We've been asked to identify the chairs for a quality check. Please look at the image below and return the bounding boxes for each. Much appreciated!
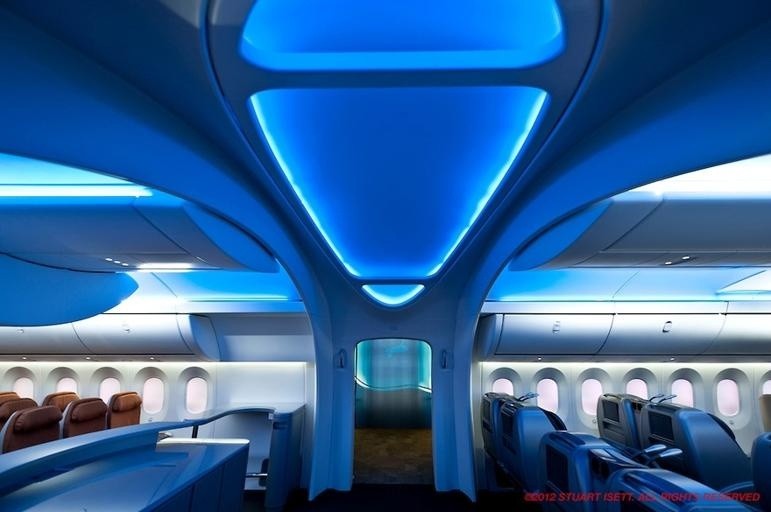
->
[0,391,142,454]
[480,393,771,512]
